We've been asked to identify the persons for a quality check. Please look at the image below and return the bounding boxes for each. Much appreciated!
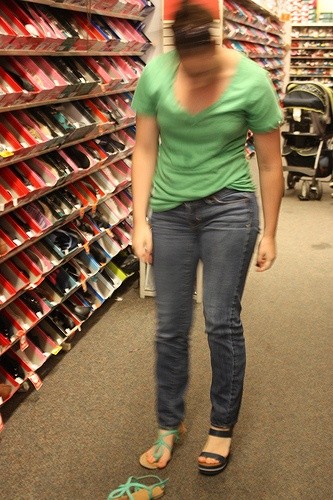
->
[130,4,285,473]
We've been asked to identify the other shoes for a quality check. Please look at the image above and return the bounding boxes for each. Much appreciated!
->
[224,1,333,160]
[0,0,151,405]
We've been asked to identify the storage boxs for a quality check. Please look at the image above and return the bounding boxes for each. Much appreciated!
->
[0,0,153,417]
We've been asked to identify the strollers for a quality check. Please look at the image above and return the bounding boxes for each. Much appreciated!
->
[279,80,333,200]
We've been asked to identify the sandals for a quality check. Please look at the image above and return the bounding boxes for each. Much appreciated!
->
[198,425,235,475]
[106,473,171,500]
[138,426,181,469]
